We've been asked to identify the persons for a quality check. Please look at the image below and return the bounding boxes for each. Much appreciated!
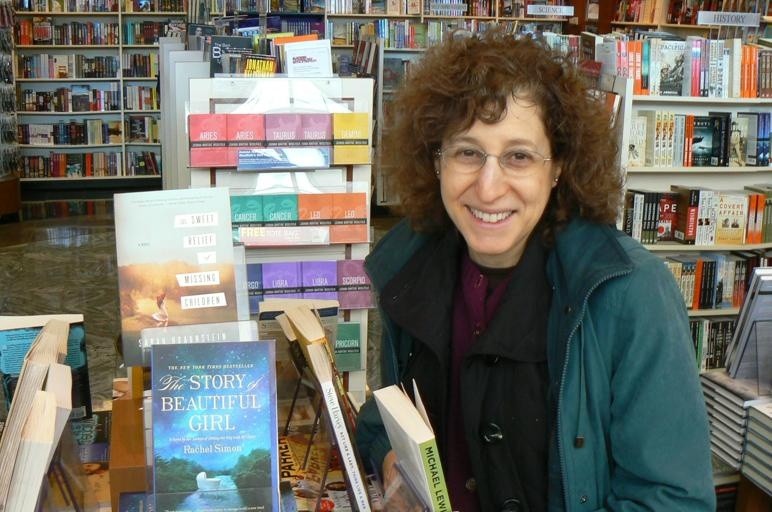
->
[362,34,717,510]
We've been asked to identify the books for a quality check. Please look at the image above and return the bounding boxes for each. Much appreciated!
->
[370,375,456,511]
[116,310,371,511]
[1,1,165,180]
[1,309,109,511]
[370,1,772,310]
[696,309,772,499]
[160,1,377,305]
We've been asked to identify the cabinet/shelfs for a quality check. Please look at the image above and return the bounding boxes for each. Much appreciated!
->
[156,0,772,427]
[0,0,185,183]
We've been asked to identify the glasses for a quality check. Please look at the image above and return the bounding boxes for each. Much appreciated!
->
[439,145,554,176]
